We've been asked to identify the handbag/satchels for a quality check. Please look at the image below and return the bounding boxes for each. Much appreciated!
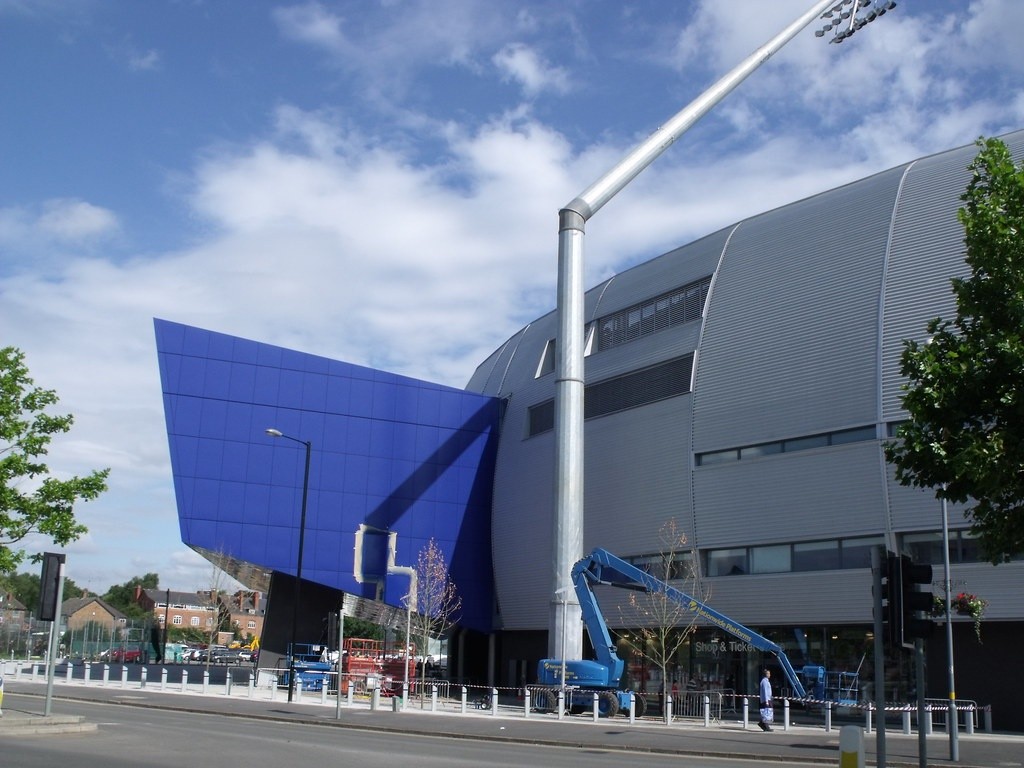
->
[760,707,774,723]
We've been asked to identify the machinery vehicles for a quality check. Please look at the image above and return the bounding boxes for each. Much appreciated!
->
[227,633,259,651]
[533,546,807,718]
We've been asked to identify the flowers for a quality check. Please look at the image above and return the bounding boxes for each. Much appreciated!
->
[929,594,955,617]
[957,586,990,647]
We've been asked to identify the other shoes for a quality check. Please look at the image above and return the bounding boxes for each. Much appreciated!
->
[764,727,774,732]
[758,721,767,731]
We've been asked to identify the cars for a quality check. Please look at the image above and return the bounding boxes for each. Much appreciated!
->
[98,644,142,662]
[181,645,259,664]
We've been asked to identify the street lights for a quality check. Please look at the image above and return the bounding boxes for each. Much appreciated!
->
[264,429,311,700]
[550,0,900,663]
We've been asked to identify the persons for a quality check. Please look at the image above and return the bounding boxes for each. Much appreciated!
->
[672,679,679,714]
[758,670,774,731]
[657,679,668,715]
[305,646,324,661]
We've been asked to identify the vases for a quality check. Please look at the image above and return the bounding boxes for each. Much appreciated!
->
[958,606,970,616]
[933,600,946,610]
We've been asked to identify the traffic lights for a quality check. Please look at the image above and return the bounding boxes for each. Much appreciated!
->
[871,550,935,653]
